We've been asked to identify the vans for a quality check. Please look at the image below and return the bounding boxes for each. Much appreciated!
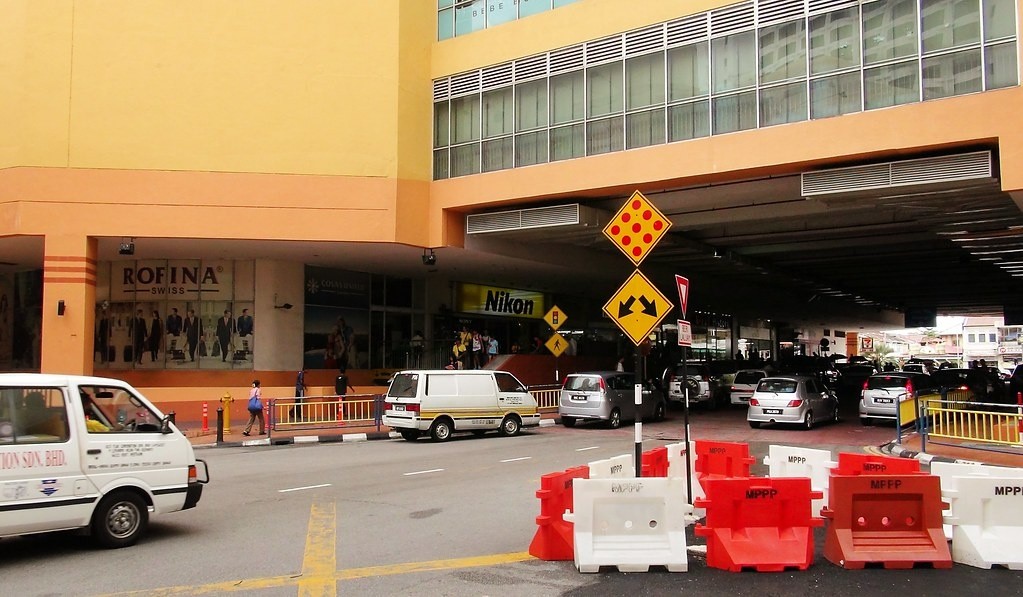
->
[0,372,209,548]
[559,371,668,429]
[381,370,541,442]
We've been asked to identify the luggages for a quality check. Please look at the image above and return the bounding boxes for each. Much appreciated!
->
[105,339,115,362]
[124,339,133,362]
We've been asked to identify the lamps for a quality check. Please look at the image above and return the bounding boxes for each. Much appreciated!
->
[58,300,66,315]
[119,237,134,255]
[422,248,436,266]
[275,303,293,309]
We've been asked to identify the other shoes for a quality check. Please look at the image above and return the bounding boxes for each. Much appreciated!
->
[242,432,250,436]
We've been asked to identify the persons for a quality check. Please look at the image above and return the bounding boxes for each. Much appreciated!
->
[147,310,163,362]
[410,331,424,368]
[237,309,254,360]
[98,309,112,364]
[335,368,355,420]
[289,367,310,418]
[324,316,354,370]
[443,325,987,420]
[183,310,204,362]
[216,310,236,362]
[166,307,182,335]
[242,380,266,436]
[0,391,137,443]
[128,310,146,368]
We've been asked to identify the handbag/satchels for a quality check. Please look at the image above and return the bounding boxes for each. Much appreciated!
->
[211,340,220,356]
[466,343,473,351]
[200,340,207,356]
[248,388,263,411]
[458,350,465,361]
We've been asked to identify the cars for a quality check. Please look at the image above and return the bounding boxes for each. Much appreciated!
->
[730,355,877,430]
[860,358,1023,429]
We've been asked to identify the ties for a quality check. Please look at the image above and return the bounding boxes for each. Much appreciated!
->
[191,318,193,325]
[225,318,227,327]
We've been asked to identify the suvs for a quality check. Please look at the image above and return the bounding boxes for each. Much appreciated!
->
[667,357,737,410]
